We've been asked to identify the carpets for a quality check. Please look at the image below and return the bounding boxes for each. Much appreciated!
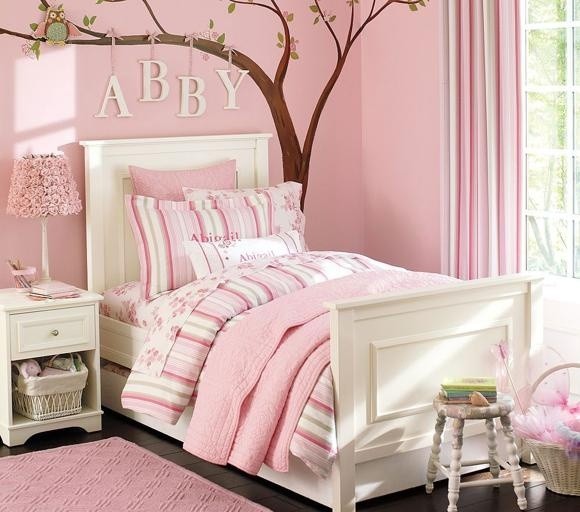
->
[0,435,275,512]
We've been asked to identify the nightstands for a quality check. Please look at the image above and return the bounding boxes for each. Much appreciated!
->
[0,282,107,447]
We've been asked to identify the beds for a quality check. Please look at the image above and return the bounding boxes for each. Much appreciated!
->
[86,267,546,511]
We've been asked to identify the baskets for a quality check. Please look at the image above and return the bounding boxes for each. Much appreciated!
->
[12,353,89,421]
[519,363,580,497]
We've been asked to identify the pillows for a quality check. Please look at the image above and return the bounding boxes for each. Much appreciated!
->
[121,151,309,302]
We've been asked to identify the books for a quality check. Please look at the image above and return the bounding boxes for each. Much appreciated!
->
[30,281,77,296]
[440,377,498,405]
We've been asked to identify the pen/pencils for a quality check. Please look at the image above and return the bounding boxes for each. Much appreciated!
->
[6,259,31,288]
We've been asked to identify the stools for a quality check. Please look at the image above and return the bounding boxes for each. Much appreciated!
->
[424,396,528,512]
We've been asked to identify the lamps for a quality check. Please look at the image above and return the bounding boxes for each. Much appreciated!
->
[5,151,83,295]
